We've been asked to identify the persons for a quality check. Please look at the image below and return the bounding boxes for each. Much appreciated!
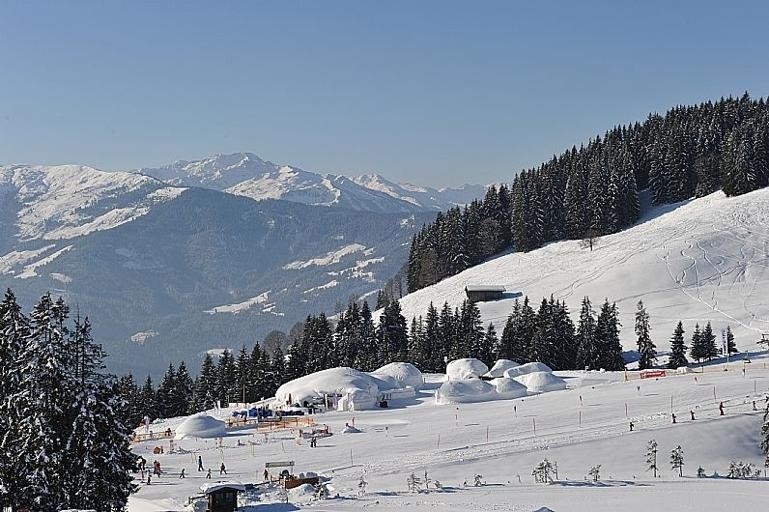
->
[206,467,212,478]
[630,422,634,430]
[136,457,163,484]
[220,462,227,475]
[310,436,314,447]
[262,468,268,480]
[180,467,186,477]
[314,436,317,447]
[197,455,205,471]
[672,413,677,423]
[719,401,726,415]
[690,410,696,420]
